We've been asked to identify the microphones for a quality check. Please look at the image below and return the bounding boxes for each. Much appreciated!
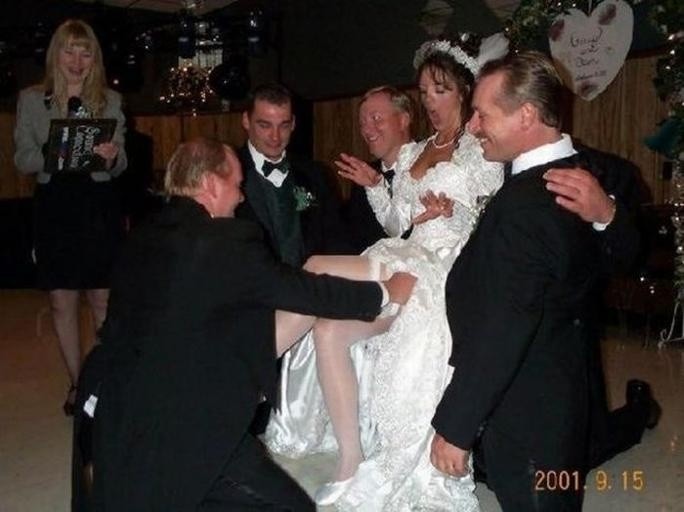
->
[67,96,82,118]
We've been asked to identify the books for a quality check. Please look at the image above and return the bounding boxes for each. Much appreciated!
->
[42,117,118,176]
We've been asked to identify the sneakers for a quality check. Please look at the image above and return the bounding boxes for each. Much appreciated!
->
[315,473,357,508]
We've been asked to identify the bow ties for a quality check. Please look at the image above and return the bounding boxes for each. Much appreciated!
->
[262,157,295,175]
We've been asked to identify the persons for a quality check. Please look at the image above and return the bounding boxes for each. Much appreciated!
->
[232,84,351,270]
[543,50,662,470]
[257,32,505,512]
[430,53,617,512]
[351,81,416,252]
[15,19,129,416]
[71,136,418,512]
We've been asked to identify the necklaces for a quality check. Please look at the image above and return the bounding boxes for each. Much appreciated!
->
[432,130,465,149]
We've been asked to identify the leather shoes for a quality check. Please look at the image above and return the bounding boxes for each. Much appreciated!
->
[627,378,663,426]
[61,385,77,418]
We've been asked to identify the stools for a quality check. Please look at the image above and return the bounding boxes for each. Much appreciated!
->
[604,272,678,352]
